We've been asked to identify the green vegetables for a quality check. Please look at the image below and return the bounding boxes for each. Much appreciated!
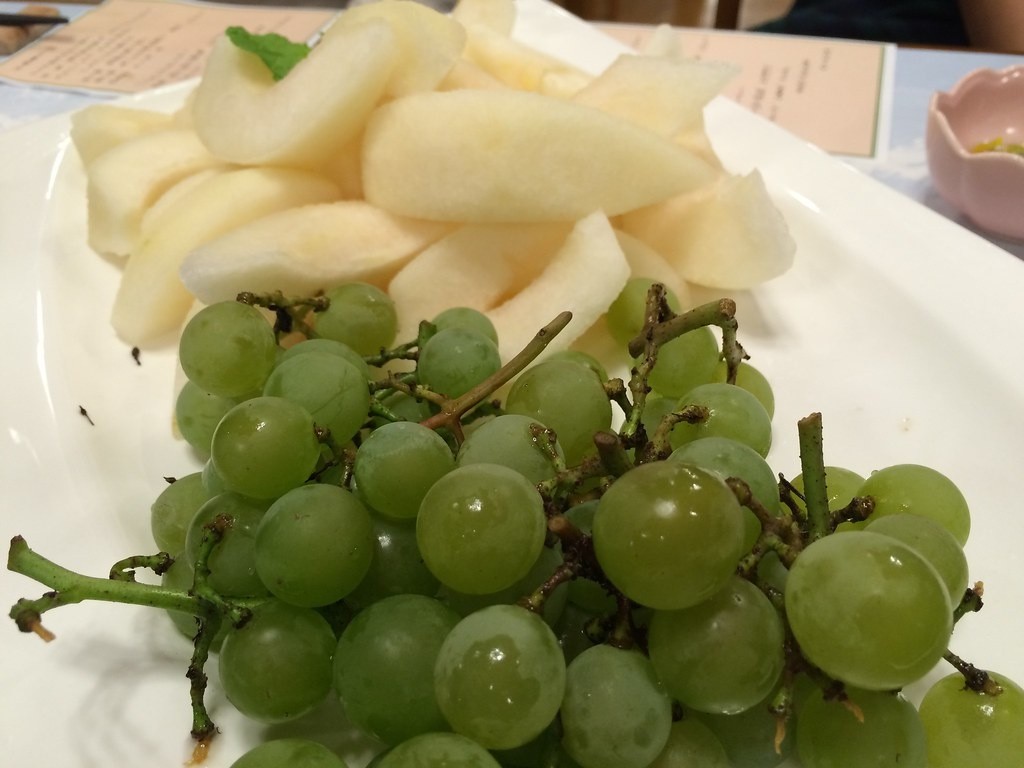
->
[226,25,309,83]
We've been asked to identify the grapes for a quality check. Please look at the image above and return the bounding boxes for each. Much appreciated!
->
[7,272,1024,768]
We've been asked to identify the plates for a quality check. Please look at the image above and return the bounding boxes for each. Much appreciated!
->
[0,0,1024,768]
